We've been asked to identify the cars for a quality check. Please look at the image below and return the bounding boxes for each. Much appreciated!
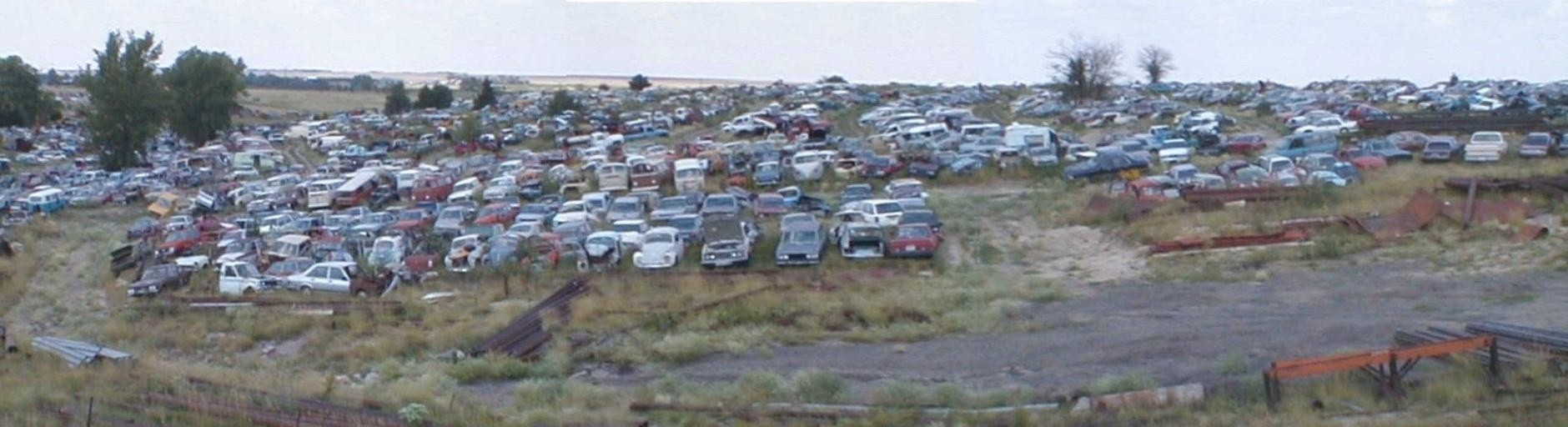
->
[0,94,118,226]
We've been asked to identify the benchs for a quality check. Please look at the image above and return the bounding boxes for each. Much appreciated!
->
[1264,333,1496,406]
